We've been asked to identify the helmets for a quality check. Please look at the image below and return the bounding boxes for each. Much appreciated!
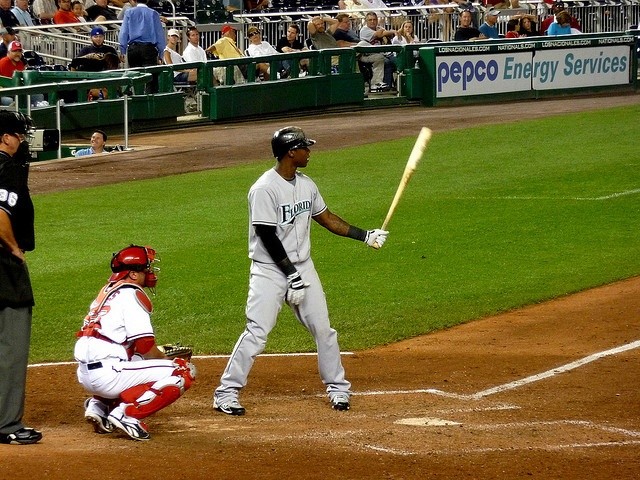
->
[271,126,316,154]
[1,111,38,154]
[108,244,160,296]
[90,28,103,35]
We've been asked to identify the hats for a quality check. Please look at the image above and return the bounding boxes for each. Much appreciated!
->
[8,41,22,51]
[485,7,501,17]
[168,29,180,38]
[222,25,235,33]
[0,26,15,35]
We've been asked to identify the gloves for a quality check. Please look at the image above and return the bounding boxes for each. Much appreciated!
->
[364,229,389,250]
[286,272,310,306]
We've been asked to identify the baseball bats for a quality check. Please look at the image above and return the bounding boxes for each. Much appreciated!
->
[373,126,433,250]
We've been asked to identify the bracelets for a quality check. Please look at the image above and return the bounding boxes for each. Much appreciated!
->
[345,225,367,242]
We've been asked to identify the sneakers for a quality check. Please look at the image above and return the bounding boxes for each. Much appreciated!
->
[213,389,245,416]
[83,397,117,434]
[1,428,41,445]
[330,387,351,410]
[371,82,387,93]
[281,65,291,79]
[108,406,150,440]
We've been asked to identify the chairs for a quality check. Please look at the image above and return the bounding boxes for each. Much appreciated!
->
[163,59,199,112]
[53,64,67,70]
[304,37,339,74]
[425,38,444,43]
[544,29,548,35]
[244,45,277,82]
[24,66,37,73]
[40,64,53,70]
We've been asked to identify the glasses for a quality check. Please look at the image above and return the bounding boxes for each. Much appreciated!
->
[187,27,196,31]
[343,21,350,23]
[12,51,21,54]
[367,19,376,21]
[248,32,258,36]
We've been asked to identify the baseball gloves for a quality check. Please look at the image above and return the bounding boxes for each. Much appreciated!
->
[162,345,193,364]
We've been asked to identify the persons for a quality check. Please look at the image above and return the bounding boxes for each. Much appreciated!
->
[68,26,117,60]
[33,0,59,19]
[118,0,166,94]
[163,29,196,85]
[454,10,487,40]
[70,1,106,23]
[212,127,390,415]
[70,53,118,72]
[0,110,43,445]
[0,26,15,59]
[338,0,371,18]
[75,130,107,158]
[392,20,420,58]
[74,245,196,441]
[205,25,272,79]
[0,41,25,77]
[334,14,389,92]
[478,7,501,39]
[276,25,309,74]
[547,11,572,35]
[505,19,528,39]
[519,15,541,36]
[360,11,401,90]
[0,0,20,34]
[540,2,580,35]
[54,0,87,32]
[247,26,307,78]
[307,17,362,74]
[183,26,218,85]
[83,0,118,30]
[10,0,34,26]
[359,0,392,25]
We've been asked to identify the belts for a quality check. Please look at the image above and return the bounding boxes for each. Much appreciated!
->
[131,43,150,47]
[87,362,103,371]
